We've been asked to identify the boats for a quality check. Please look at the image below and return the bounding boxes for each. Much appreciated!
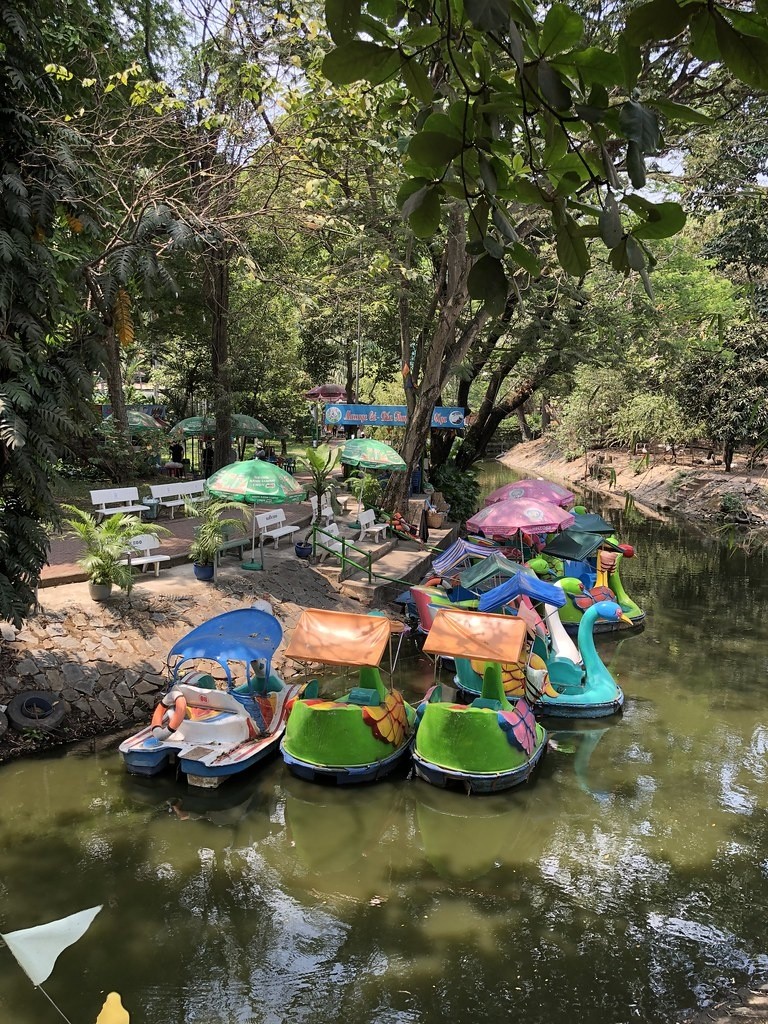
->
[414,609,550,796]
[115,607,301,789]
[409,478,647,720]
[285,606,420,787]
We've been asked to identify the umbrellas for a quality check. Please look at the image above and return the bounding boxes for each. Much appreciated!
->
[305,384,347,404]
[485,479,574,546]
[202,457,307,562]
[169,416,216,437]
[103,410,162,445]
[466,494,575,565]
[338,437,407,521]
[231,413,271,438]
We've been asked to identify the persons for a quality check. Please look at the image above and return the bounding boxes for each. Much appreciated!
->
[169,441,184,477]
[254,441,265,457]
[204,442,214,479]
[332,425,336,435]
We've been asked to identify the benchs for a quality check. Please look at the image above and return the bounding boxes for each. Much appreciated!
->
[150,479,214,520]
[255,508,301,550]
[193,522,250,567]
[358,509,390,543]
[318,523,354,565]
[309,494,336,527]
[89,487,150,524]
[91,533,170,577]
[326,490,349,515]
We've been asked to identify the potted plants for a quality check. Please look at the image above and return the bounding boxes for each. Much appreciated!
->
[178,492,255,581]
[45,503,175,601]
[295,444,347,559]
